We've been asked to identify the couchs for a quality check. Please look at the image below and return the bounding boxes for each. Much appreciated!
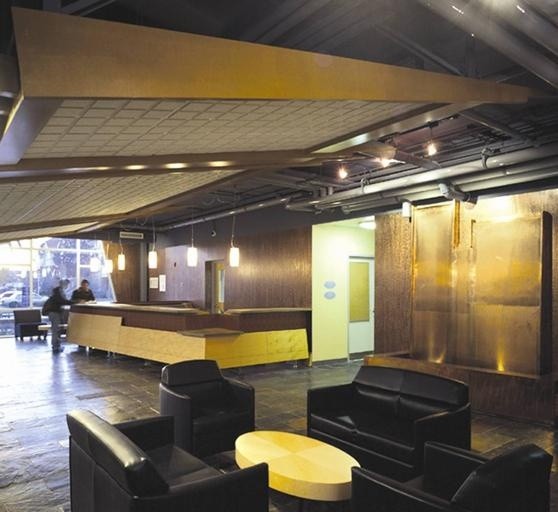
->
[159,360,256,458]
[352,441,553,512]
[13,310,48,341]
[306,365,472,484]
[66,410,269,512]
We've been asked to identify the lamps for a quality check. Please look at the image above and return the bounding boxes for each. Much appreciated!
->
[187,204,198,267]
[377,133,400,168]
[426,123,438,156]
[148,215,158,269]
[229,194,239,268]
[338,163,348,179]
[117,229,126,271]
[358,221,376,231]
[103,231,113,273]
[90,231,100,272]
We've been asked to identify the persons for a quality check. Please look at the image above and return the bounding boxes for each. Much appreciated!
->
[71,280,96,303]
[42,287,78,352]
